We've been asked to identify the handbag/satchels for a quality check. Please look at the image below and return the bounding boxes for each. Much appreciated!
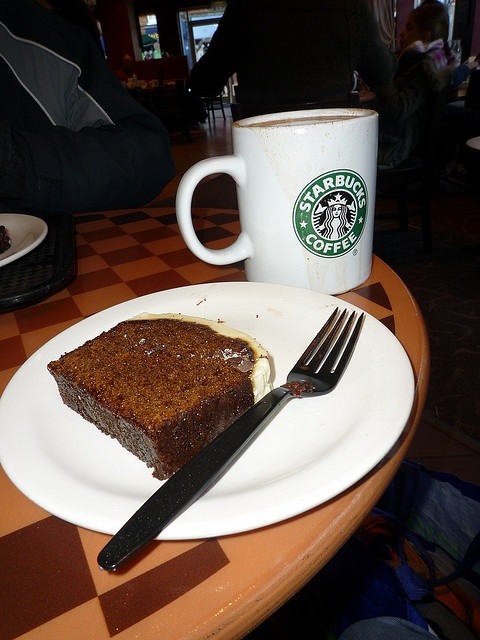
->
[375,456,480,586]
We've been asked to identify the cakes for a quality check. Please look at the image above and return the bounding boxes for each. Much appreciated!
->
[0,220,12,256]
[43,309,274,482]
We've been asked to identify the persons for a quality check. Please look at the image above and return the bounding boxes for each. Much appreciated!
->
[359,0,447,201]
[110,53,133,84]
[433,54,480,191]
[181,0,394,109]
[1,2,177,213]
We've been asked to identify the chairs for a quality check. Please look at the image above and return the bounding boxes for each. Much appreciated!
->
[376,79,448,252]
[231,92,364,122]
[201,93,226,124]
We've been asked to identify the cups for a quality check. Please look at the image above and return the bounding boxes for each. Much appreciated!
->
[176,107,380,296]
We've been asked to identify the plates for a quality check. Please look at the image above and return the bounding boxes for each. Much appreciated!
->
[1,212,48,268]
[0,281,415,542]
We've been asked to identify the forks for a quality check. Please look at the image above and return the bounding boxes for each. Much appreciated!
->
[97,308,364,568]
